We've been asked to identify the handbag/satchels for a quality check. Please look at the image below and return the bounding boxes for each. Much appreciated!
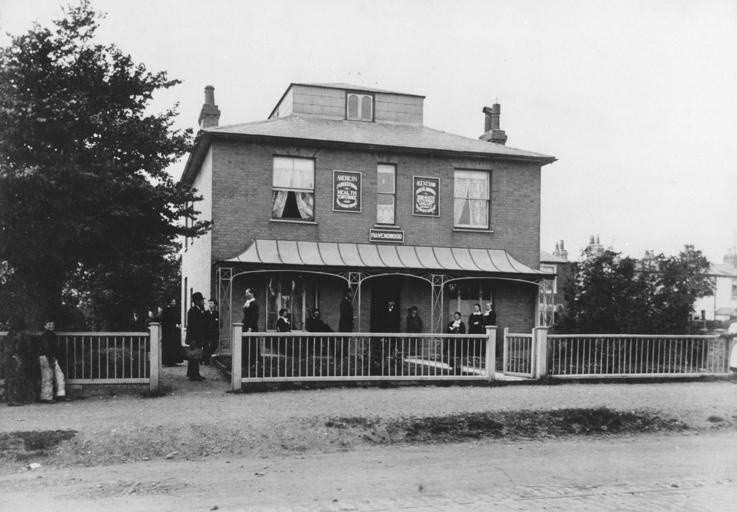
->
[183,345,203,360]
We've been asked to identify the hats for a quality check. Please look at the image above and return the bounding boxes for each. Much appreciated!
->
[191,292,205,302]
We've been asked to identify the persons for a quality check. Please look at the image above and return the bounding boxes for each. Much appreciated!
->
[240,287,354,370]
[161,292,223,380]
[34,316,73,403]
[385,298,423,355]
[444,303,497,359]
[3,317,32,407]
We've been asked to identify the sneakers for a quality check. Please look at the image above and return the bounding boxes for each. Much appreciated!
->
[190,373,205,381]
[41,399,55,402]
[57,395,71,402]
